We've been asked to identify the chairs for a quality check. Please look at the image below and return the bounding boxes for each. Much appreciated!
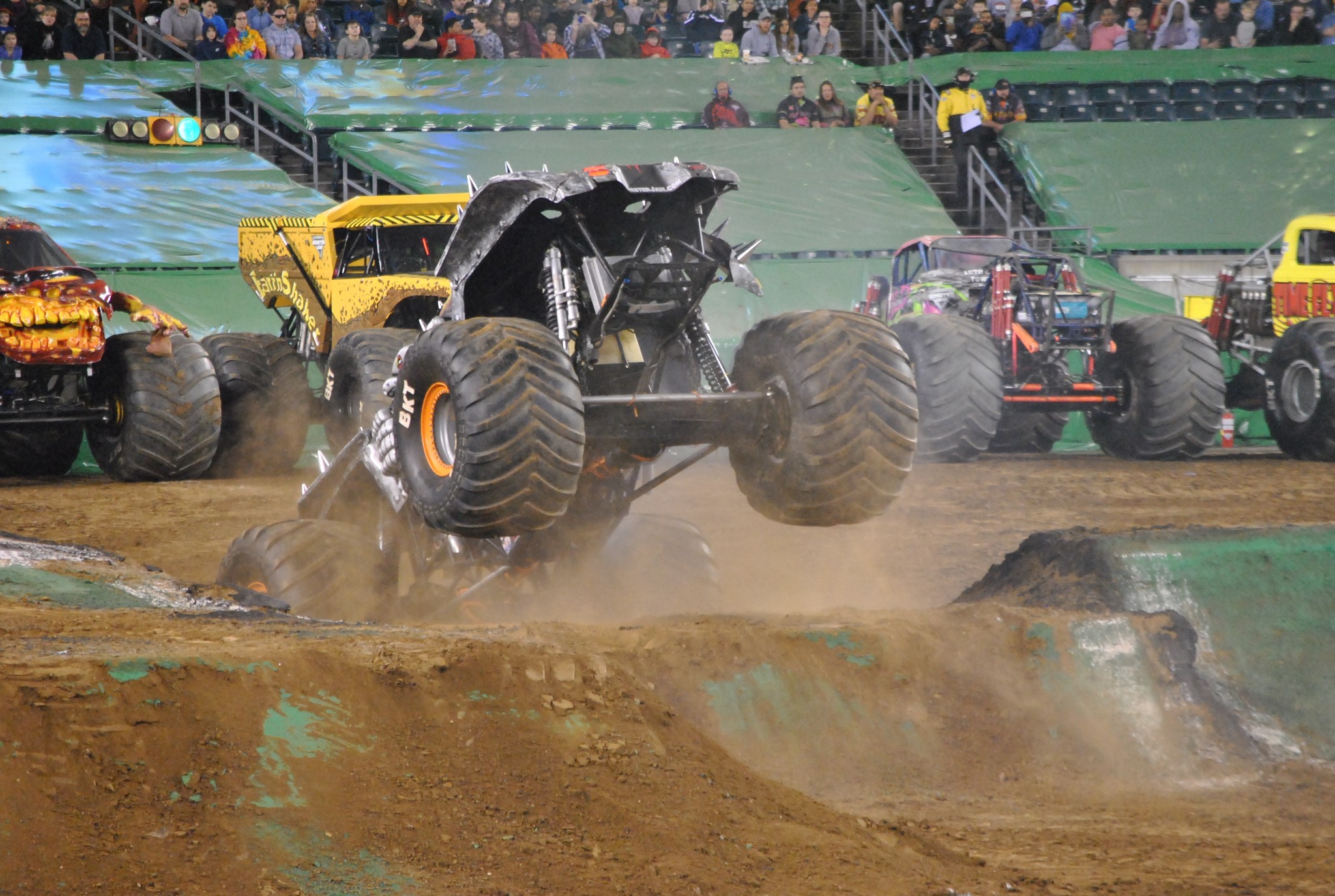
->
[317,1,399,60]
[627,24,718,59]
[980,76,1335,123]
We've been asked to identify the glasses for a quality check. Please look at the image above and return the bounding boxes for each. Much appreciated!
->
[816,16,831,20]
[870,82,883,87]
[271,14,286,19]
[236,17,246,21]
[577,12,586,15]
[347,25,360,30]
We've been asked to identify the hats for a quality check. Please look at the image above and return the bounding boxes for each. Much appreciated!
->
[408,7,422,17]
[957,67,971,75]
[576,4,590,13]
[869,80,884,88]
[995,79,1011,89]
[758,12,771,20]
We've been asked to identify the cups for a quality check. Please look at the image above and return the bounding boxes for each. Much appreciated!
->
[446,38,457,52]
[742,49,750,58]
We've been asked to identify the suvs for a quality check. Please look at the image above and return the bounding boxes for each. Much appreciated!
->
[849,232,1228,467]
[1195,211,1334,464]
[0,216,220,483]
[212,157,925,625]
[197,191,479,475]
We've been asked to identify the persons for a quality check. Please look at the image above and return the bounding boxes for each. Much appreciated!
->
[776,76,822,128]
[855,81,898,127]
[702,82,751,128]
[936,66,996,208]
[0,0,1335,62]
[982,78,1026,157]
[814,81,851,128]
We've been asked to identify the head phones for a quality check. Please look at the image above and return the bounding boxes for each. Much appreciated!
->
[713,88,732,96]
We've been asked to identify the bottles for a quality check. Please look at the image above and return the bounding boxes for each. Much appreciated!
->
[399,17,404,27]
[887,128,895,140]
[1127,19,1138,32]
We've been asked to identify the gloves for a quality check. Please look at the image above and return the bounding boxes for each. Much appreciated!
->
[942,131,954,149]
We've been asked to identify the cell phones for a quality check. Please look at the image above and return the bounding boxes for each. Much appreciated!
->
[578,16,582,23]
